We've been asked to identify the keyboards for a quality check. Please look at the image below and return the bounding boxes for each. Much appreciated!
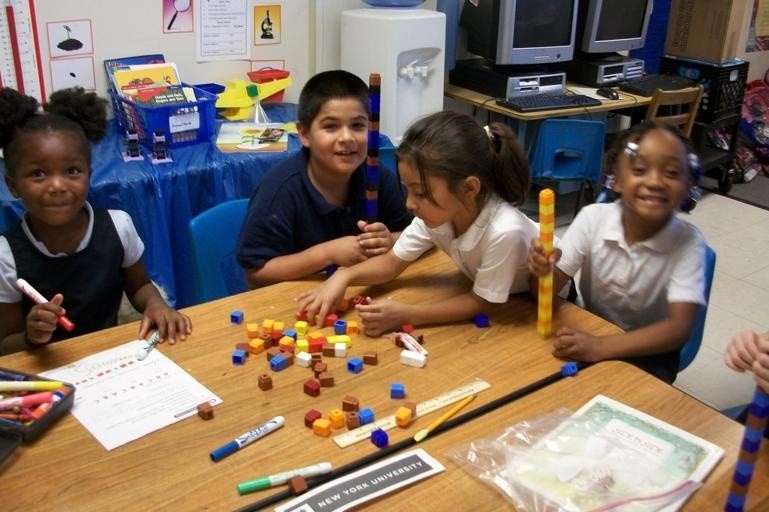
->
[495,94,601,112]
[618,74,696,96]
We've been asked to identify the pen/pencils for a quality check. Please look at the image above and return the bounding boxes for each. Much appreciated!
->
[413,395,477,442]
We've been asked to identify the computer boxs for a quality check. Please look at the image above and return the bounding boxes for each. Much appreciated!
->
[567,55,645,87]
[454,58,567,101]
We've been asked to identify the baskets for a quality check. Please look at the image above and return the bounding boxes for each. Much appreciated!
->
[107,82,219,150]
[661,56,749,123]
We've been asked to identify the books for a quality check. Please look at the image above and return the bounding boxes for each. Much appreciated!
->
[104,53,199,144]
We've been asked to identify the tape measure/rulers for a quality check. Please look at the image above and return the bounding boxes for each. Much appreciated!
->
[332,376,492,449]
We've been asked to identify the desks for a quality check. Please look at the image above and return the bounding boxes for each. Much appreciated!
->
[2,100,412,310]
[256,359,769,511]
[2,247,627,512]
[442,69,697,207]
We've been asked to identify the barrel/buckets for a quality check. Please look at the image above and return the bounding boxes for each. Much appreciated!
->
[361,0,428,9]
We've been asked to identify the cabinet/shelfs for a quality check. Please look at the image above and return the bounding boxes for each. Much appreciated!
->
[660,55,749,176]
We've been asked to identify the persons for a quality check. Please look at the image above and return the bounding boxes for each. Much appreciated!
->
[291,108,579,338]
[723,328,769,441]
[524,117,708,387]
[0,84,193,356]
[234,68,416,291]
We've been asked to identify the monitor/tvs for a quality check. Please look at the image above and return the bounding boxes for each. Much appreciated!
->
[580,0,654,64]
[458,0,579,73]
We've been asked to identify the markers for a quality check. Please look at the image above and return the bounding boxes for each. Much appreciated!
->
[15,278,75,332]
[137,328,161,361]
[0,369,71,427]
[237,462,332,496]
[210,415,286,462]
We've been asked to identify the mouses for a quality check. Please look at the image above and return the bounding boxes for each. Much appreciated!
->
[596,87,618,100]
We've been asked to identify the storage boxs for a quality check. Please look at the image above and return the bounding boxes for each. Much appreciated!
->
[663,0,746,64]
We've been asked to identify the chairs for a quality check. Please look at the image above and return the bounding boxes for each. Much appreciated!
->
[524,118,607,217]
[643,83,705,139]
[675,242,716,372]
[188,196,251,303]
